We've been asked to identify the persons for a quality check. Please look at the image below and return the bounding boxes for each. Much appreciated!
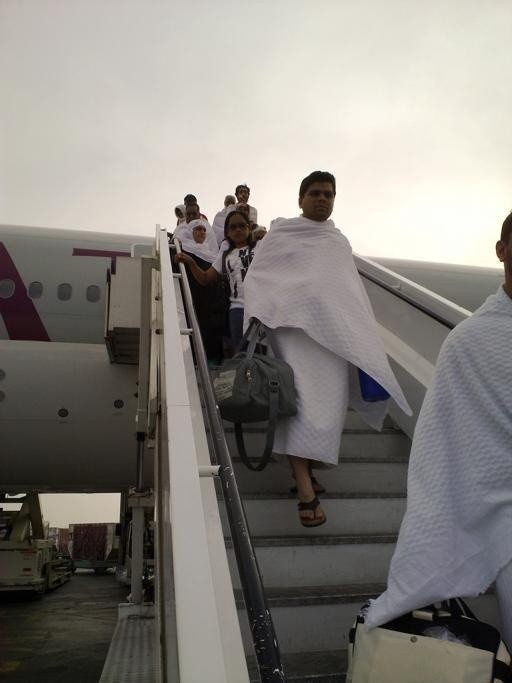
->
[168,185,272,370]
[241,170,412,528]
[366,209,512,683]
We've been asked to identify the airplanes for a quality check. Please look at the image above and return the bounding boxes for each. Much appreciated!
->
[0,224,512,681]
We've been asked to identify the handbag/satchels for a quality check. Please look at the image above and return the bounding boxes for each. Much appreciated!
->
[343,592,512,682]
[213,317,299,424]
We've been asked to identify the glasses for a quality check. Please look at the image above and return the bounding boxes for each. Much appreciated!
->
[228,222,250,230]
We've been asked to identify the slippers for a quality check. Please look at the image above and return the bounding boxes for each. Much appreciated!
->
[290,475,326,493]
[297,492,327,527]
